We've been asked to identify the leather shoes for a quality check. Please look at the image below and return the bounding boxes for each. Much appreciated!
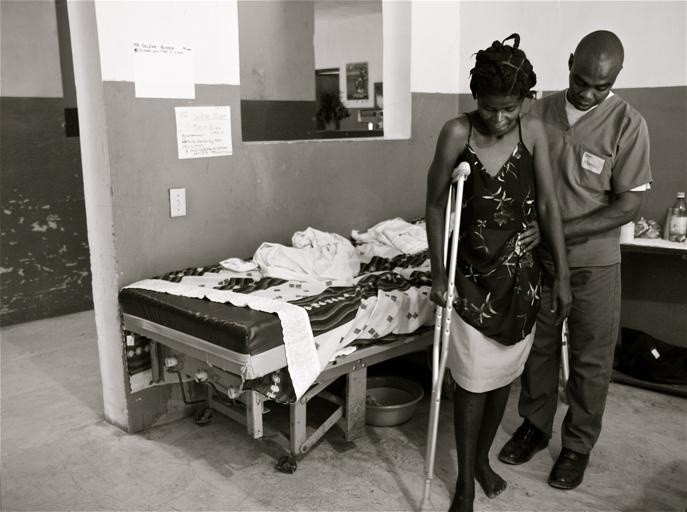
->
[497,422,551,466]
[545,447,591,488]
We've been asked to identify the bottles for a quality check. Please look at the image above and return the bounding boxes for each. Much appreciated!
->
[669,192,685,242]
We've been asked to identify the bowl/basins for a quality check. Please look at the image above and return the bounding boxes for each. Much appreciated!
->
[364,378,423,428]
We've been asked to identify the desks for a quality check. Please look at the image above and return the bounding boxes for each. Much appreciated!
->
[609,239,687,399]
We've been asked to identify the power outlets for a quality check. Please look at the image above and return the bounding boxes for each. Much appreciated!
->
[169,188,186,218]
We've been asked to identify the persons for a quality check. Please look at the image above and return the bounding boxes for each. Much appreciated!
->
[426,34,573,512]
[497,31,653,490]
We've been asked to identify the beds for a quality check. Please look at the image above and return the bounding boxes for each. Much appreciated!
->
[118,216,441,474]
